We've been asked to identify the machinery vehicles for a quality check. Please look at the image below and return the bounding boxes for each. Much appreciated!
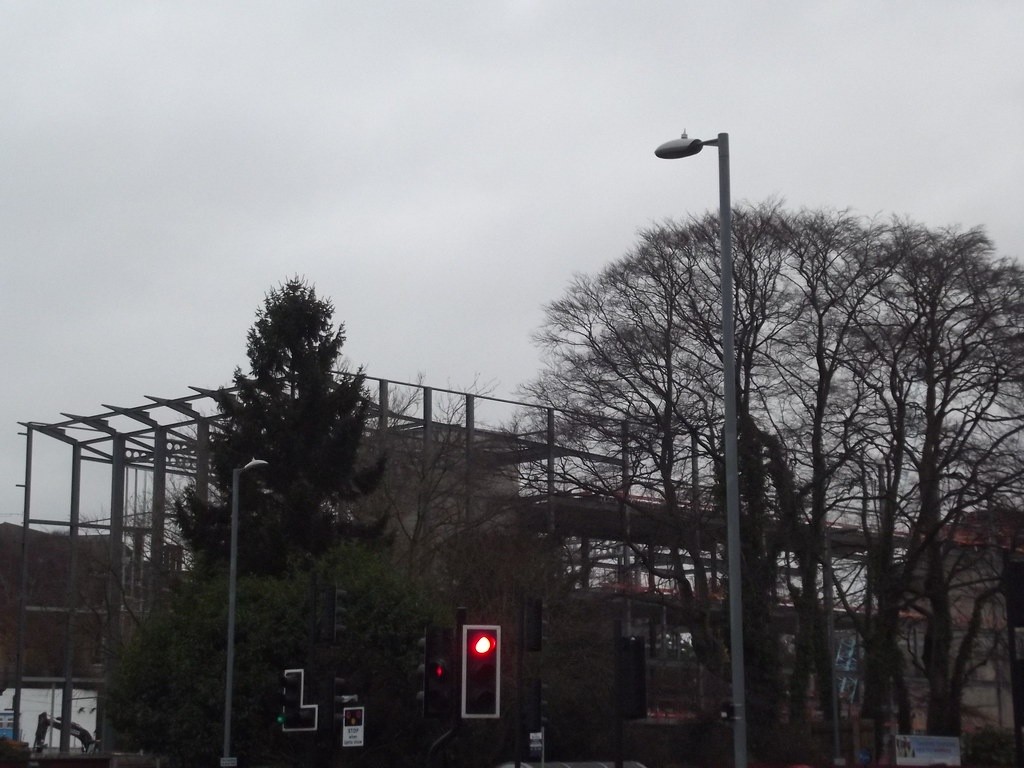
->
[34,712,98,758]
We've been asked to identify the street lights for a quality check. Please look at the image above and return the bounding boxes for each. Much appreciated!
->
[220,456,269,768]
[655,126,755,768]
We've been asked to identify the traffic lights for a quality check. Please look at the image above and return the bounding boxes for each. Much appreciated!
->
[322,586,348,640]
[424,624,457,722]
[278,668,347,737]
[461,622,502,719]
